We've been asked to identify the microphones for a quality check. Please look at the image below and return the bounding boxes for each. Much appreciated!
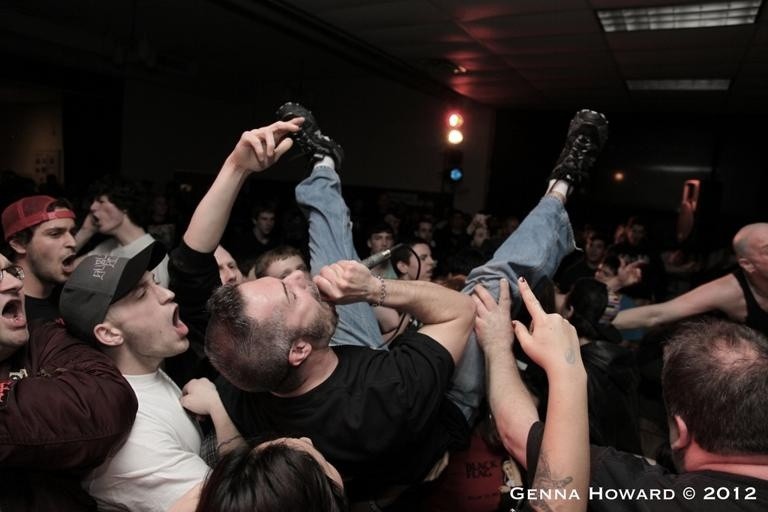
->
[316,242,407,296]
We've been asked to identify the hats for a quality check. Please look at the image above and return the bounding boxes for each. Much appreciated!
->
[59,240,165,340]
[2,198,77,238]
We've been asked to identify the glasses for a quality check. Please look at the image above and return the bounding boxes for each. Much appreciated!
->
[1,266,24,280]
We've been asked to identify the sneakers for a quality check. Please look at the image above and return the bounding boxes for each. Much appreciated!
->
[278,102,344,167]
[549,109,608,192]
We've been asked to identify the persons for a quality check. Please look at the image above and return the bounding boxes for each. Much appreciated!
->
[0,102,768,512]
